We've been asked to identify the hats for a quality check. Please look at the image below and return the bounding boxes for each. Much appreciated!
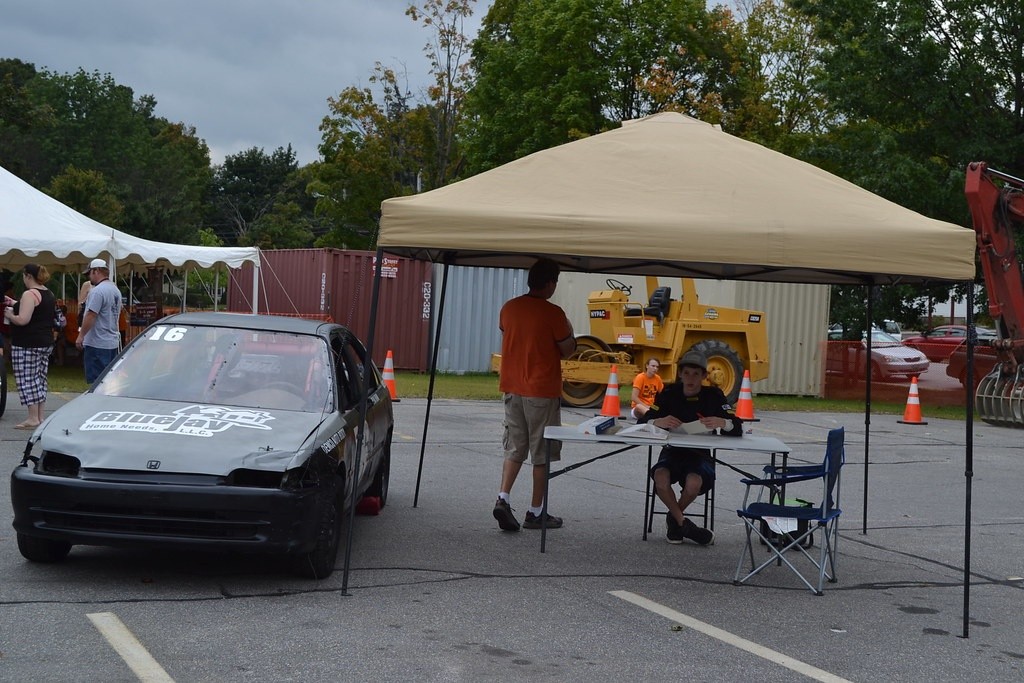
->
[81,259,108,277]
[675,350,709,372]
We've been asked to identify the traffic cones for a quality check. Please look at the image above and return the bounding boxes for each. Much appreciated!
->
[896,376,928,426]
[383,349,401,403]
[731,368,760,423]
[595,364,627,420]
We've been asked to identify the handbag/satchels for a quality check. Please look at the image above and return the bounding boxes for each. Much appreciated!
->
[52,298,66,333]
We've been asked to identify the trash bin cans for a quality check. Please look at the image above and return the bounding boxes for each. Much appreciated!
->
[129,312,149,342]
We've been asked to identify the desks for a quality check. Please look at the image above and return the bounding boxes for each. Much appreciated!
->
[541,425,792,566]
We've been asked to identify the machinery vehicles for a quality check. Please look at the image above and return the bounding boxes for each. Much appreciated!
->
[491,277,770,409]
[965,162,1024,426]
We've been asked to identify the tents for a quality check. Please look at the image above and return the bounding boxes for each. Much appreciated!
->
[340,112,977,640]
[0,165,306,342]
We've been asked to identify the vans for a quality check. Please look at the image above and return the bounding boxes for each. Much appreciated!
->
[827,319,902,342]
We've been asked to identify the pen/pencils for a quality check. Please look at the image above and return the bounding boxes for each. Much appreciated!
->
[697,413,704,419]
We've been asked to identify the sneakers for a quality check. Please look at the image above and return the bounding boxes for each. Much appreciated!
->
[665,510,684,545]
[678,519,715,546]
[521,508,563,530]
[493,496,524,532]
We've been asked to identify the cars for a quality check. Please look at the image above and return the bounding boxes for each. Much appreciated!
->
[827,328,930,380]
[10,310,394,580]
[122,298,139,307]
[901,325,990,362]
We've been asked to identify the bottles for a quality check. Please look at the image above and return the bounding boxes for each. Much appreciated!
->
[3,305,13,325]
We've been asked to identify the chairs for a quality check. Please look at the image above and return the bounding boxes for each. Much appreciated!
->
[626,286,671,317]
[733,426,846,595]
[647,448,716,542]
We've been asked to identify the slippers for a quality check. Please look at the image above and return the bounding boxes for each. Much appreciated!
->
[14,421,38,430]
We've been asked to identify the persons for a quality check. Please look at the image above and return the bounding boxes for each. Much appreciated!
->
[74,259,122,384]
[636,351,743,546]
[0,263,56,430]
[630,357,664,420]
[493,258,578,532]
